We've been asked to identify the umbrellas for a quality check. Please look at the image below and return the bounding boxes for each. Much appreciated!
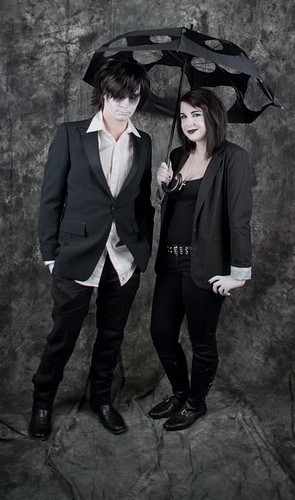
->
[80,25,286,193]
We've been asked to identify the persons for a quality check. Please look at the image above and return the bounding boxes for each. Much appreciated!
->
[28,58,156,442]
[149,88,252,430]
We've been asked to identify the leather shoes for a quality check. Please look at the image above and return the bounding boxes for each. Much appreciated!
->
[148,390,207,432]
[87,394,127,435]
[28,403,53,441]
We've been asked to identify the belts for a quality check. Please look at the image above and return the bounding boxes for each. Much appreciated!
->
[165,244,192,257]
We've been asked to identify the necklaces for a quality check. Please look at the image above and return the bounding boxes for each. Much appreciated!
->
[178,148,205,190]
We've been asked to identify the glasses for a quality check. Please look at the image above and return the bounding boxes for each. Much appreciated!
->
[108,90,141,105]
